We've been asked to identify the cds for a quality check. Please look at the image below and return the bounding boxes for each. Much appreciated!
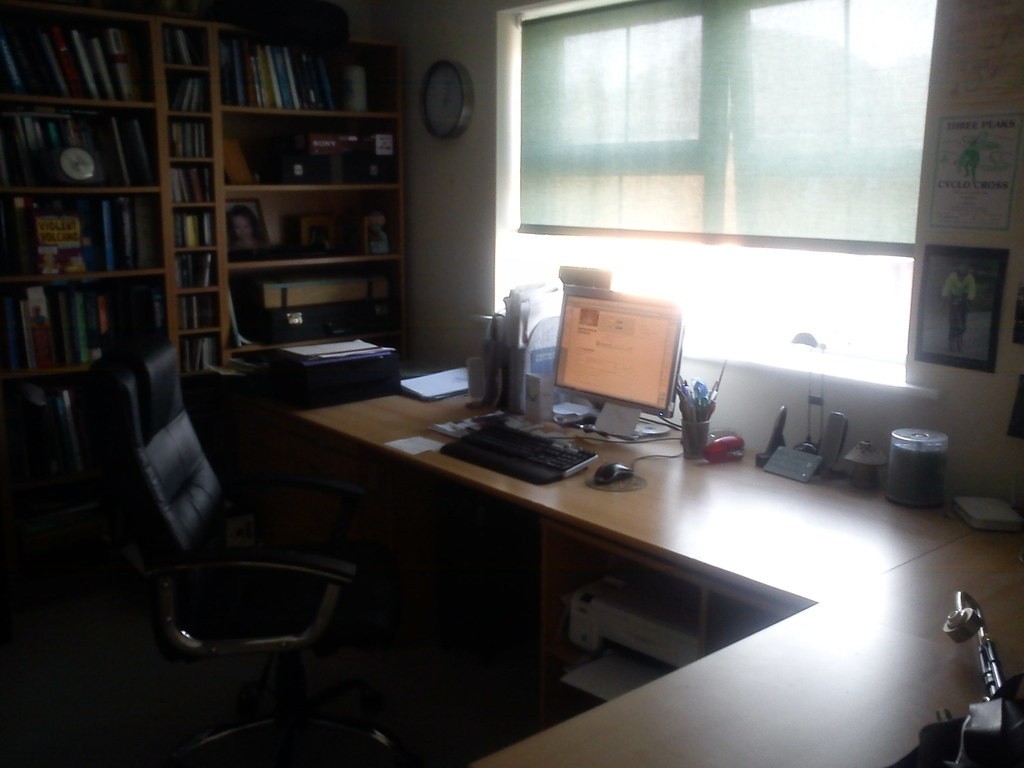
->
[635,423,670,434]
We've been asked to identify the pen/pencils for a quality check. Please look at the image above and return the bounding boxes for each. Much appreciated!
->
[676,363,726,424]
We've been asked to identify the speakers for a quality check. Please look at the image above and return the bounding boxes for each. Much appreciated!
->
[818,412,848,480]
[755,405,789,465]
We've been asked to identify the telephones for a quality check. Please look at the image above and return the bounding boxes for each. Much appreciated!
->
[755,401,788,473]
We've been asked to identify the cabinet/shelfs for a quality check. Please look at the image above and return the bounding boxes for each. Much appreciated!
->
[1,0,408,645]
[538,515,709,730]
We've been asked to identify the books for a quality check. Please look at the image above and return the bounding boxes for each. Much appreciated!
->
[219,37,368,112]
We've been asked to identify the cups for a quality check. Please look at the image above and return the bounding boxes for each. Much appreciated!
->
[681,419,711,459]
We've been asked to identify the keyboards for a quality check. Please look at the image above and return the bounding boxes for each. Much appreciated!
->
[438,423,598,486]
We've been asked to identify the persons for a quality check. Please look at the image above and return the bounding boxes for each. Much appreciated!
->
[941,263,976,352]
[0,27,217,371]
[227,205,268,259]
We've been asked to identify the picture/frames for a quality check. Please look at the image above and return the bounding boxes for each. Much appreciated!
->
[914,244,1010,373]
[224,199,268,250]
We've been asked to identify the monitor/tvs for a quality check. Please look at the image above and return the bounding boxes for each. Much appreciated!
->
[554,285,687,442]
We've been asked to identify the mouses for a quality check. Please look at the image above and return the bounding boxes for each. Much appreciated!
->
[595,462,634,486]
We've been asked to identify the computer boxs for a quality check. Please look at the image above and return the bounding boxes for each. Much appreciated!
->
[433,485,542,661]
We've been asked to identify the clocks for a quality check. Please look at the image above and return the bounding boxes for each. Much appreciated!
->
[418,60,474,139]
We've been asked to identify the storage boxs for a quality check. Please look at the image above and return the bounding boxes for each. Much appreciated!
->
[261,132,397,157]
[231,346,402,409]
[524,372,554,423]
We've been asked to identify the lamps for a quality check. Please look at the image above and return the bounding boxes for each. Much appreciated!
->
[791,333,824,455]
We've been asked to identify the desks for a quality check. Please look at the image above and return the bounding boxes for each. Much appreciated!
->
[234,358,1024,768]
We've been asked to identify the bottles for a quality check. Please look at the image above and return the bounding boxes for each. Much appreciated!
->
[884,426,951,507]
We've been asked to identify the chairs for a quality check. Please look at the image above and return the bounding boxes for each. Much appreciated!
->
[81,332,426,767]
